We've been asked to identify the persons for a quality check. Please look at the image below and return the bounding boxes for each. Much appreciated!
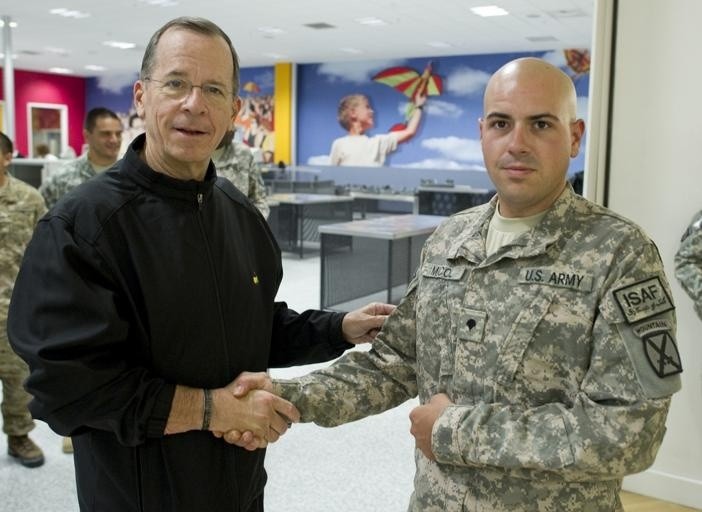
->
[1,133,46,468]
[235,95,274,151]
[210,119,271,222]
[38,145,59,185]
[209,58,686,512]
[6,17,401,512]
[38,108,129,455]
[675,211,701,319]
[325,86,430,172]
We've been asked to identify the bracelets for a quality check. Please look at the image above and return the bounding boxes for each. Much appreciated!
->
[198,384,213,438]
[414,103,425,111]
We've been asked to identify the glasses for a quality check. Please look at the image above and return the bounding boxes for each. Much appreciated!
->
[144,77,234,107]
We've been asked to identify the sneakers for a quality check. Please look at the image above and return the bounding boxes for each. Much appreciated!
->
[61,435,76,455]
[7,433,45,469]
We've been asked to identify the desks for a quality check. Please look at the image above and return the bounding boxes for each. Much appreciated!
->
[260,164,495,259]
[319,215,452,311]
